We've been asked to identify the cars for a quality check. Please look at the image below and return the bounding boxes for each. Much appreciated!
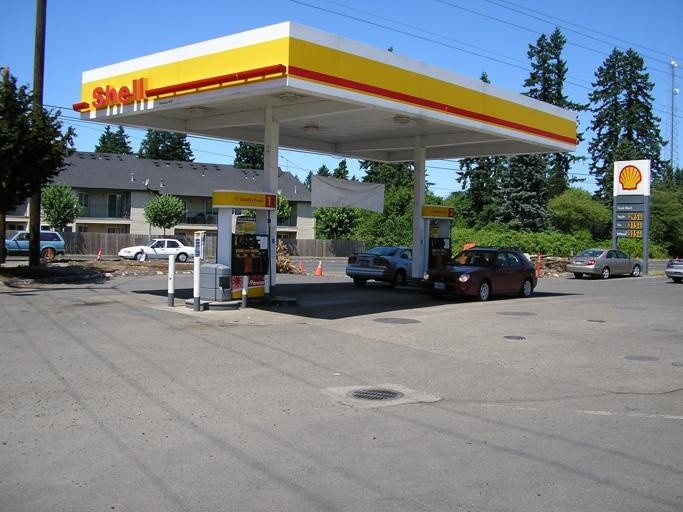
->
[664,258,683,283]
[423,245,538,301]
[117,238,195,263]
[345,245,413,286]
[565,248,642,279]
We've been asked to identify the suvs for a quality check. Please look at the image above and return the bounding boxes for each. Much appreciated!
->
[4,230,67,260]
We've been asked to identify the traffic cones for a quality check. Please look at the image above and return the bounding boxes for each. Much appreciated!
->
[314,259,323,277]
